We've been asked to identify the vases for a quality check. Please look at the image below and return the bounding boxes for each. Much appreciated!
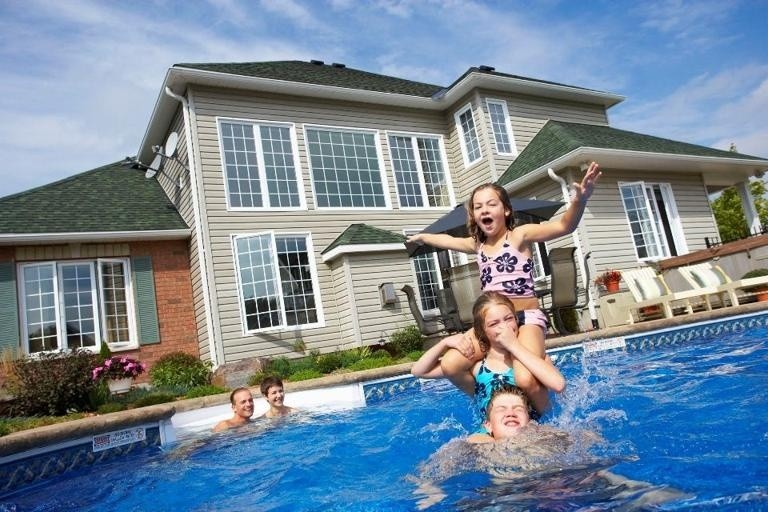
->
[604,281,620,293]
[104,376,134,394]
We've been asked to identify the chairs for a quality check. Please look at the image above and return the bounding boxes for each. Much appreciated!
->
[536,246,593,337]
[401,261,482,337]
[622,261,767,321]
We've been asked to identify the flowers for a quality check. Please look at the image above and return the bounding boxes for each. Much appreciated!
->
[91,354,146,384]
[595,267,621,285]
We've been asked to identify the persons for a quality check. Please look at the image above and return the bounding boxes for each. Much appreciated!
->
[404,160,604,417]
[257,377,317,427]
[172,386,259,458]
[410,291,566,439]
[406,382,692,512]
